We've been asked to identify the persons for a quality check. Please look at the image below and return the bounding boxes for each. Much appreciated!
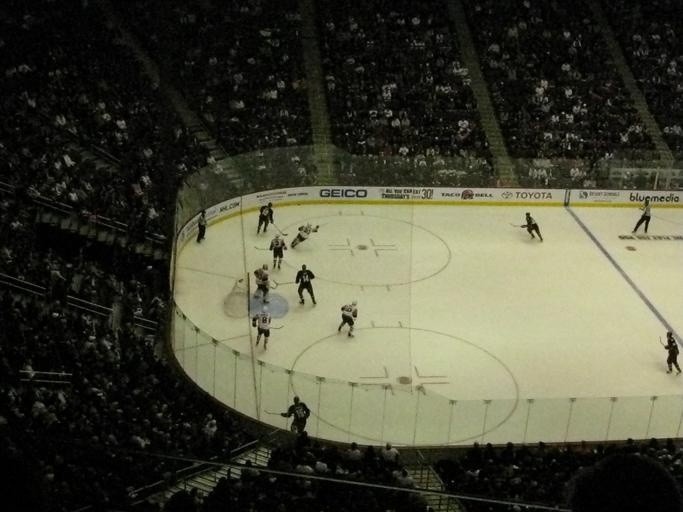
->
[0,189,227,512]
[336,299,358,338]
[268,234,287,269]
[255,201,275,233]
[294,264,317,306]
[251,304,273,350]
[251,263,271,304]
[280,395,311,433]
[227,409,683,511]
[290,220,320,250]
[632,199,652,233]
[664,331,682,374]
[0,0,683,191]
[520,212,543,241]
